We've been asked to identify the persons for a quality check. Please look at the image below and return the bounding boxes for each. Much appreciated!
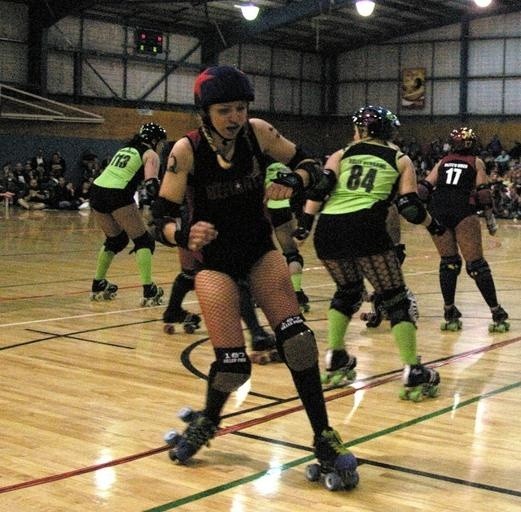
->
[88,123,168,307]
[360,127,521,323]
[289,106,446,387]
[147,65,359,472]
[162,160,311,335]
[0,148,112,212]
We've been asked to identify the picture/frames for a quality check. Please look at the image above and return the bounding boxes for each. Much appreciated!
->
[400,67,425,111]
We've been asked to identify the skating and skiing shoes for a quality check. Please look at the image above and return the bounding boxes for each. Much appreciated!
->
[489,304,510,333]
[164,406,218,465]
[248,334,285,365]
[361,290,381,327]
[319,349,356,387]
[398,356,440,402]
[140,284,202,333]
[441,305,462,331]
[295,289,310,313]
[90,278,118,300]
[305,429,359,491]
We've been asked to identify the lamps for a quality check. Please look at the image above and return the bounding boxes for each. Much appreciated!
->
[355,0,375,17]
[241,6,260,21]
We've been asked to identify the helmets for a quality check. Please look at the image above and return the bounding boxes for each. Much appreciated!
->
[194,65,255,108]
[352,105,401,142]
[140,122,167,144]
[447,125,477,153]
